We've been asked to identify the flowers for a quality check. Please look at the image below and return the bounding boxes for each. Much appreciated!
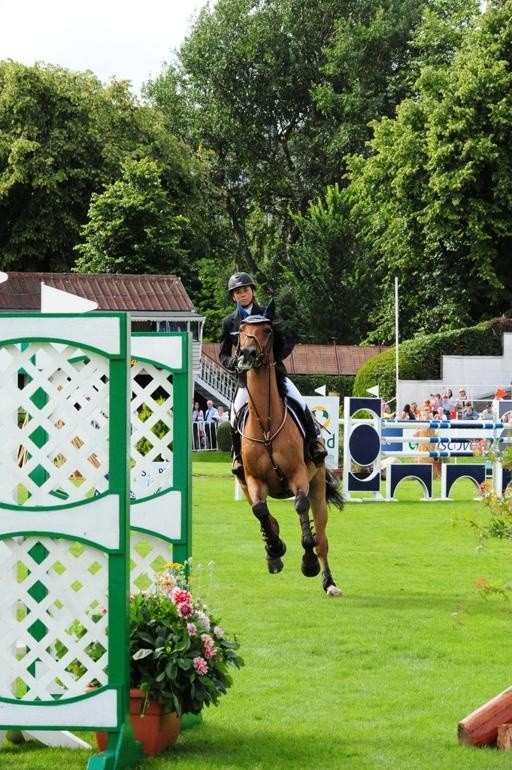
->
[54,557,245,720]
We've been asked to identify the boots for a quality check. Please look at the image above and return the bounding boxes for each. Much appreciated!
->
[227,424,243,475]
[303,404,327,465]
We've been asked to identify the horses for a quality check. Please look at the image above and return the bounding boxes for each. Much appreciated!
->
[231,298,349,599]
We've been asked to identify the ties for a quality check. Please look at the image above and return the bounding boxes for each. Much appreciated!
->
[205,410,211,421]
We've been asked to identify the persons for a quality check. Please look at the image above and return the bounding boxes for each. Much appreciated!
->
[215,273,328,474]
[204,400,218,449]
[380,386,497,420]
[216,405,229,427]
[193,402,205,450]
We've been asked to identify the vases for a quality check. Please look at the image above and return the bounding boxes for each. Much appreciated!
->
[81,685,181,756]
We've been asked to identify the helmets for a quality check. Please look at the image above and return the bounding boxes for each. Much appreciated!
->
[227,272,257,294]
